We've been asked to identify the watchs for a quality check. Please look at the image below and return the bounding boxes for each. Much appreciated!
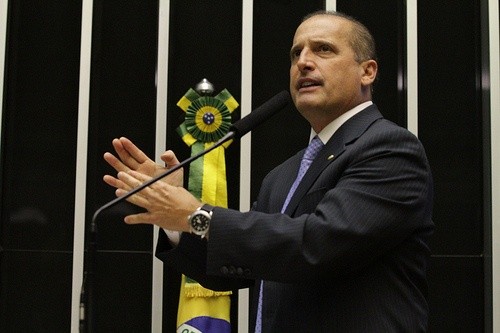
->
[186,202,213,240]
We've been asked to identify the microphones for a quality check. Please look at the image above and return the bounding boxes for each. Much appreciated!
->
[90,88,291,250]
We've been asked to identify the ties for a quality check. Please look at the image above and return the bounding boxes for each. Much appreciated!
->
[254,137,322,332]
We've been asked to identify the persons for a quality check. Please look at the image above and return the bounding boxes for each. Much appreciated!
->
[102,10,433,333]
